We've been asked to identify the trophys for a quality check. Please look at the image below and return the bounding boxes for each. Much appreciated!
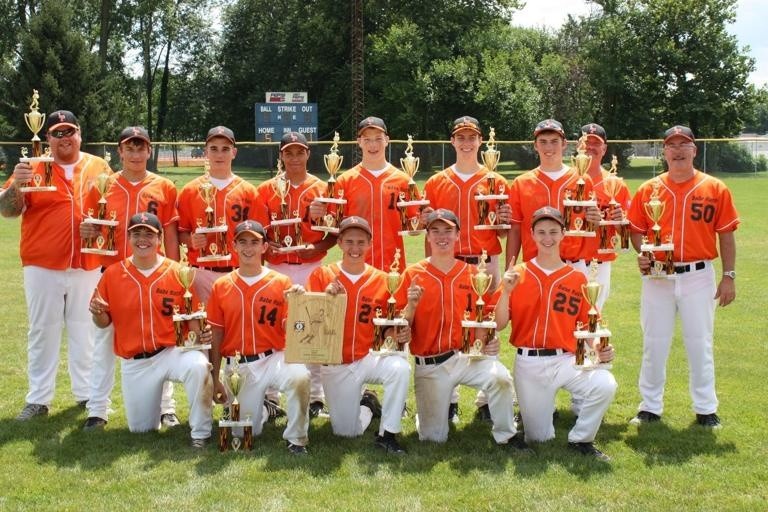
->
[573,257,612,369]
[598,153,630,254]
[171,243,213,350]
[474,125,512,231]
[370,248,410,357]
[17,87,58,192]
[216,350,254,452]
[79,152,120,256]
[461,248,497,360]
[396,131,431,236]
[312,130,348,234]
[194,159,232,262]
[269,158,306,253]
[563,131,597,238]
[640,175,678,281]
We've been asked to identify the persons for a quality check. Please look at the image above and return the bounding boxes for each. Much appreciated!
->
[627,124,740,431]
[82,127,182,428]
[489,205,619,463]
[572,123,633,346]
[90,215,216,449]
[303,216,413,454]
[177,127,270,359]
[502,118,597,282]
[388,209,533,456]
[420,116,510,294]
[257,132,332,417]
[309,116,435,274]
[206,220,310,454]
[1,110,116,421]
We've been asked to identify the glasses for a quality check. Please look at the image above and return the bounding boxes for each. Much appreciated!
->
[52,127,76,138]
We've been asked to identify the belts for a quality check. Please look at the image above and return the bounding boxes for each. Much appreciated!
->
[411,348,465,366]
[226,347,287,366]
[129,344,169,362]
[452,253,495,267]
[640,260,712,273]
[515,344,578,357]
[191,264,242,273]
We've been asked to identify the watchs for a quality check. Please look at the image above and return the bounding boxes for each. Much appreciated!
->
[723,270,736,279]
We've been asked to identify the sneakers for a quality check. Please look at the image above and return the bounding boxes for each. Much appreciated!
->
[696,409,724,430]
[480,403,492,425]
[82,416,106,433]
[161,388,407,461]
[16,401,52,424]
[569,439,608,461]
[504,436,536,459]
[448,400,457,421]
[627,413,660,427]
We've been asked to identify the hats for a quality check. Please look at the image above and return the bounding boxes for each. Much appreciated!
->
[205,126,236,146]
[123,212,162,234]
[533,118,567,138]
[116,125,152,146]
[46,108,82,131]
[356,116,391,139]
[450,115,483,137]
[234,217,266,241]
[426,208,462,232]
[337,216,374,238]
[531,206,564,229]
[579,122,610,148]
[278,131,312,151]
[662,125,697,146]
